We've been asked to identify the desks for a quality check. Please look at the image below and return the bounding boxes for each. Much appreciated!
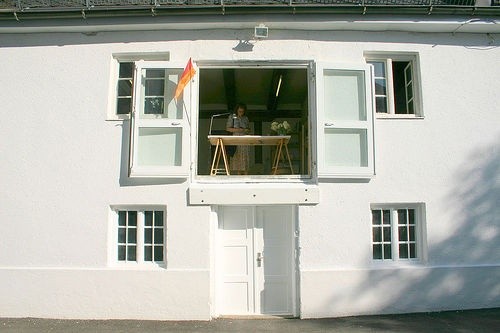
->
[207,135,294,175]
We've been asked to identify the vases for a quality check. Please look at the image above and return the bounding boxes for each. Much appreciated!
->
[279,130,287,134]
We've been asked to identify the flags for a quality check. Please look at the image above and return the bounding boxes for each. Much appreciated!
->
[174,58,195,100]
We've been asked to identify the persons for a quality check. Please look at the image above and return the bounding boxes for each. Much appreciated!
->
[226,102,251,175]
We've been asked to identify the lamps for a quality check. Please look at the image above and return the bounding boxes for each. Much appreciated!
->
[255,23,268,37]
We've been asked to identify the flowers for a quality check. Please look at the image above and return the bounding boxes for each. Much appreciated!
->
[270,121,293,134]
